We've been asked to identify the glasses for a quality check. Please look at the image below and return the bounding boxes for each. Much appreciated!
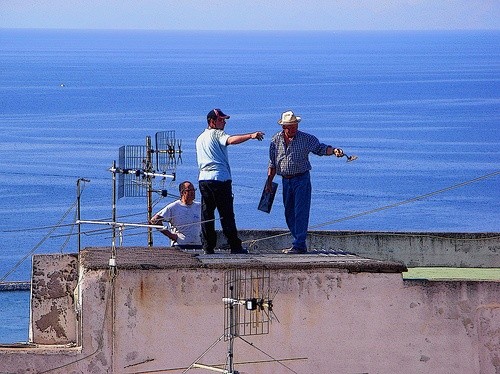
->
[183,188,197,191]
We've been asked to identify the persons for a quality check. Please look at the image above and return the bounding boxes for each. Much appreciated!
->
[267,110,345,254]
[195,108,265,254]
[150,181,201,250]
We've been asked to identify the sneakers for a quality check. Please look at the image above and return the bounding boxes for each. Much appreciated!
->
[230,247,247,254]
[282,246,307,253]
[203,249,214,254]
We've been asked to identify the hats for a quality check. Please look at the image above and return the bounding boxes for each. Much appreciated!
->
[277,110,301,125]
[206,109,230,119]
